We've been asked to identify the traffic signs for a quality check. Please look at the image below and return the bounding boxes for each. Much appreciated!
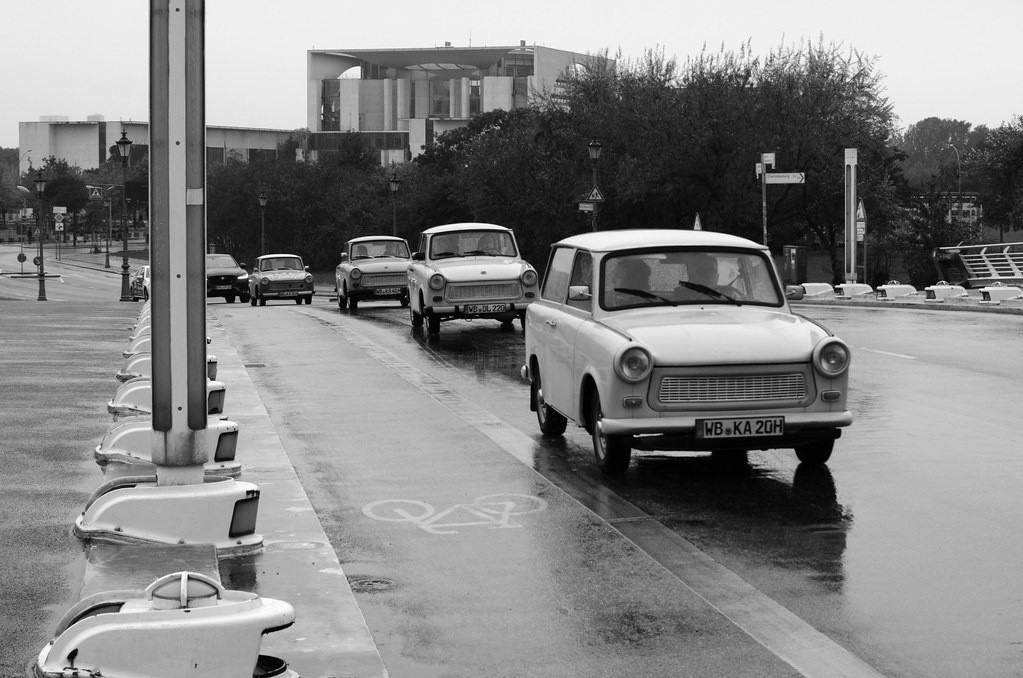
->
[765,172,805,183]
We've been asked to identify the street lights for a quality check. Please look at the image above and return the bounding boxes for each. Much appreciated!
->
[586,136,604,232]
[388,170,402,237]
[115,129,135,302]
[33,171,48,301]
[949,144,962,223]
[258,192,270,255]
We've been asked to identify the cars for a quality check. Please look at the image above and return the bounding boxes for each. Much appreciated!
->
[335,236,418,309]
[206,253,251,303]
[406,221,541,334]
[520,228,854,477]
[248,253,316,306]
[129,266,151,302]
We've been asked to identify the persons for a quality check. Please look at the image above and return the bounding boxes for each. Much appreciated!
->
[285,260,294,268]
[385,243,395,255]
[607,259,651,306]
[677,255,723,301]
[265,261,272,269]
[479,235,496,253]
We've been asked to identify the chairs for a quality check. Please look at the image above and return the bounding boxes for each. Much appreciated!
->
[611,260,652,294]
[264,260,275,270]
[357,245,367,256]
